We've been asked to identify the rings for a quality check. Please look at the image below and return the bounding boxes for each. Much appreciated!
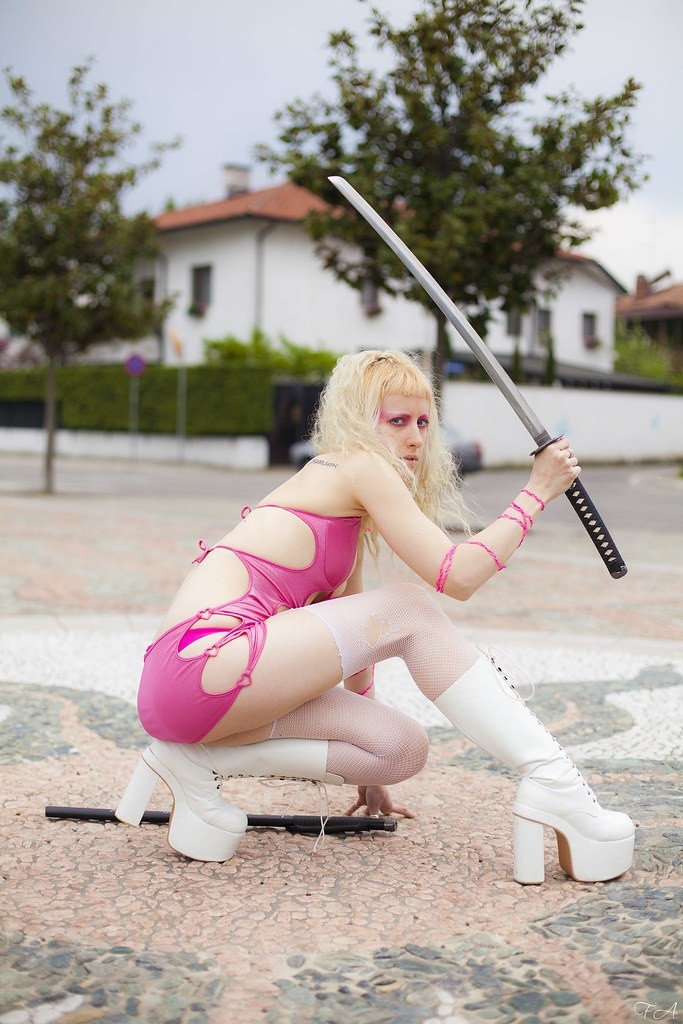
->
[565,448,572,460]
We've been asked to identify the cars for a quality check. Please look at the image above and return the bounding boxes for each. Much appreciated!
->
[286,419,482,479]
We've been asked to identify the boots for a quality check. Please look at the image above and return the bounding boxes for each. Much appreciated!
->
[433,636,635,884]
[115,738,344,862]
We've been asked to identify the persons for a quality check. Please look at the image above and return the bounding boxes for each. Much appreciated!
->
[112,345,637,886]
